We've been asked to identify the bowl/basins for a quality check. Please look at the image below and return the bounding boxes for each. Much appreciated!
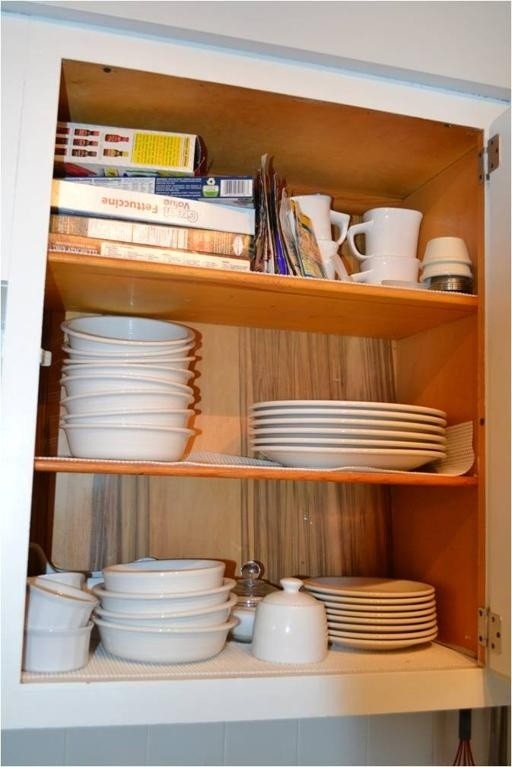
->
[22,621,94,671]
[26,577,97,630]
[90,556,241,663]
[37,573,86,589]
[58,312,199,461]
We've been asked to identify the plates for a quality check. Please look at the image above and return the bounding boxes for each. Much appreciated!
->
[247,397,448,473]
[300,573,440,650]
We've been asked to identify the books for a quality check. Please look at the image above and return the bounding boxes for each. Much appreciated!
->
[49,213,255,260]
[50,179,255,236]
[64,175,255,210]
[48,232,251,272]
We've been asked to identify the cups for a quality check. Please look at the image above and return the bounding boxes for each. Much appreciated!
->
[316,236,338,278]
[420,236,471,266]
[292,194,349,244]
[347,207,424,260]
[419,261,473,281]
[350,258,417,286]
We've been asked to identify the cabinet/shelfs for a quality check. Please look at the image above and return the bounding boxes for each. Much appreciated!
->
[3,1,510,731]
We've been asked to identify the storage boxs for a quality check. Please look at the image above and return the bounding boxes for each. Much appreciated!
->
[52,121,205,178]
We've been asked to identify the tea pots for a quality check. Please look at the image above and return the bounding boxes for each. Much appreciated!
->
[250,576,329,666]
